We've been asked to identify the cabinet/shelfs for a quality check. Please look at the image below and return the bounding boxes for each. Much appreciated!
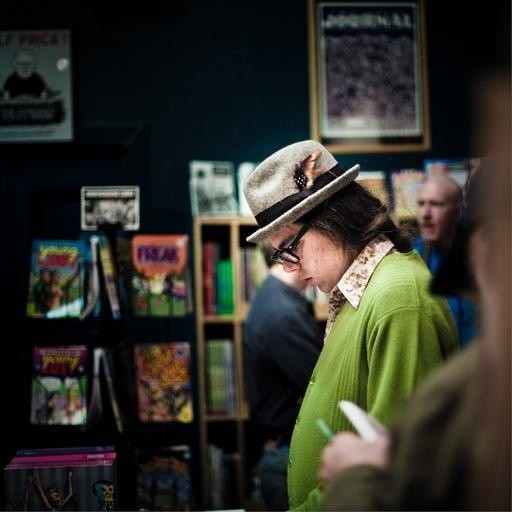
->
[193,216,331,511]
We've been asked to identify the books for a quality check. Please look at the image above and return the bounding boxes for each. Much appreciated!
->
[240,163,333,302]
[354,158,482,242]
[1,234,238,511]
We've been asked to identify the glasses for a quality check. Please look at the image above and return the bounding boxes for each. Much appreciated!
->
[271,222,312,265]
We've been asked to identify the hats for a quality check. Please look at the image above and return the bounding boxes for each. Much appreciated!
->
[243,139,360,244]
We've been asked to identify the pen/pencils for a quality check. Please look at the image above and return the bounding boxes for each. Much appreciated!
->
[316,417,334,442]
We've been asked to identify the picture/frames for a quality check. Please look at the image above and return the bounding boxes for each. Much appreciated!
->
[308,0,429,154]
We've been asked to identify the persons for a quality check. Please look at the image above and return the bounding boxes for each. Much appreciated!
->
[411,173,480,348]
[316,142,511,512]
[244,140,462,512]
[241,241,323,511]
